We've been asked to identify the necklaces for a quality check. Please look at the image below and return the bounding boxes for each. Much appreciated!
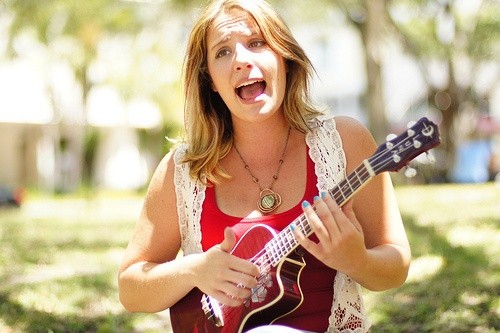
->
[233,125,292,214]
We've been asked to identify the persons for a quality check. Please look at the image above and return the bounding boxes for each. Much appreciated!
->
[117,0,411,333]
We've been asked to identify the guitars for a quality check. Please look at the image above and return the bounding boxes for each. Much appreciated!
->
[169,116,442,333]
